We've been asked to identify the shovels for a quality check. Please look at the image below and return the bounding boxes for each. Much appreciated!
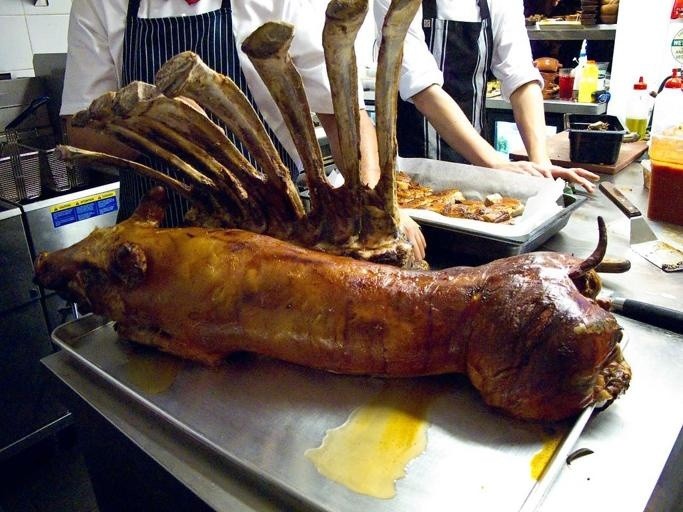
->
[599,181,682,273]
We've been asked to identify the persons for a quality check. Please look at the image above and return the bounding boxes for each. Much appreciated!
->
[372,1,600,194]
[57,1,380,228]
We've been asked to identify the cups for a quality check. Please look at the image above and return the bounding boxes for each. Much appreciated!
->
[558,69,575,100]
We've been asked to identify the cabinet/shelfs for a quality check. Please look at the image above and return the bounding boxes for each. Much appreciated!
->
[477,0,623,116]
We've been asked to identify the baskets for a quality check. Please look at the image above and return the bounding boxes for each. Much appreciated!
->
[21,127,89,192]
[0,129,42,203]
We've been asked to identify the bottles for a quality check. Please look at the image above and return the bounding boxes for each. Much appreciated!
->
[646,68,683,222]
[624,76,650,140]
[578,59,600,106]
